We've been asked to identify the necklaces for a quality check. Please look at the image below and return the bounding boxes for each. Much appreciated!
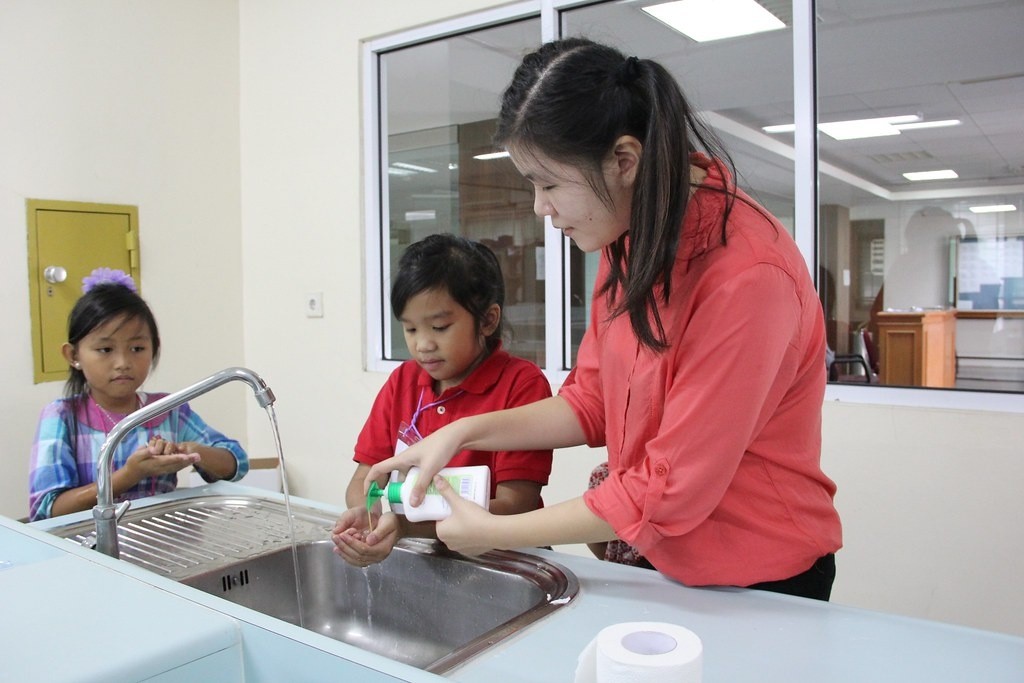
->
[96,403,117,427]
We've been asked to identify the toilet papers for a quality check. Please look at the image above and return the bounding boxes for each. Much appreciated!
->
[572,622,703,683]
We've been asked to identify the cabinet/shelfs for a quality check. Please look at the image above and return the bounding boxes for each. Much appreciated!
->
[877,311,956,388]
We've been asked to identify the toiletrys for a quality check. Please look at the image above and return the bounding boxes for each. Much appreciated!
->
[365,465,490,522]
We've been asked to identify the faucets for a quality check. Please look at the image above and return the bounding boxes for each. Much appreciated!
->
[92,367,276,557]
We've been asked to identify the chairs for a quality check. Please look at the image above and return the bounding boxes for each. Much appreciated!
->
[822,346,873,384]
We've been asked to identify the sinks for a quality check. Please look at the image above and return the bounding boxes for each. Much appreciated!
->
[170,532,580,675]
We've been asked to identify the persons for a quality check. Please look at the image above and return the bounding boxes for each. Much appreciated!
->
[28,268,250,523]
[586,462,654,569]
[332,233,554,568]
[363,37,844,602]
